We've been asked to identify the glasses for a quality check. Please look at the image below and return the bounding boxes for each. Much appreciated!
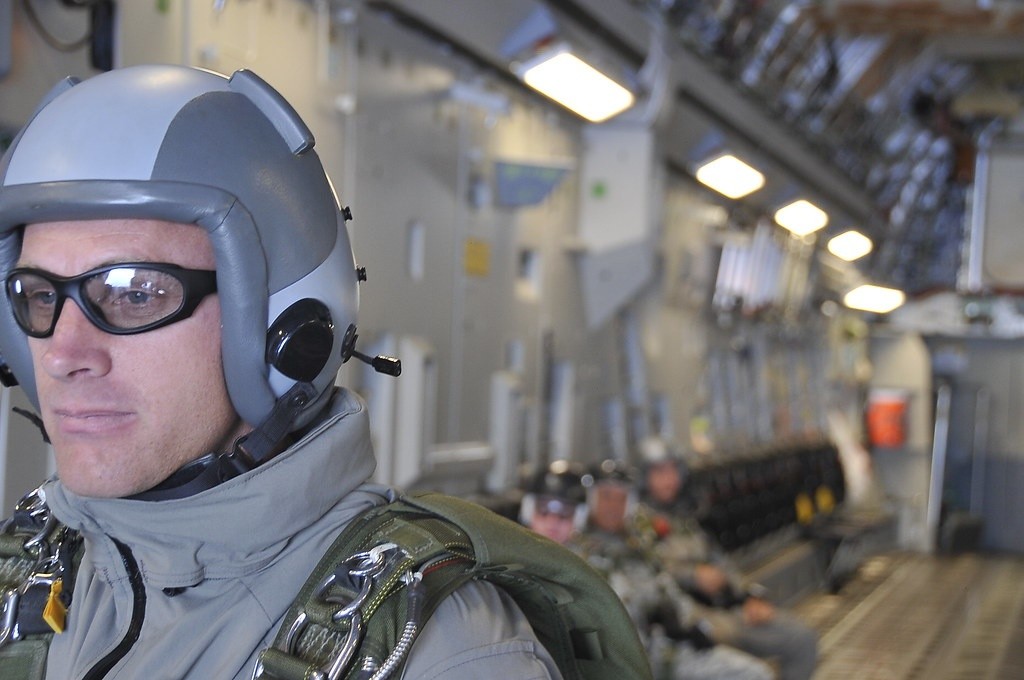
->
[4,264,217,338]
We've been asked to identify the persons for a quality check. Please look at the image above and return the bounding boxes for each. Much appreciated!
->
[0,62,653,680]
[519,434,818,680]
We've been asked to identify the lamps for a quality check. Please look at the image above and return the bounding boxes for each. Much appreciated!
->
[770,185,830,236]
[689,132,769,197]
[500,13,645,121]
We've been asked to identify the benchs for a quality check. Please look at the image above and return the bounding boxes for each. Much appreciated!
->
[685,442,899,616]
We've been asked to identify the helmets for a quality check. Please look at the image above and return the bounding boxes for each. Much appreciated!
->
[637,435,688,488]
[522,461,590,531]
[584,461,644,521]
[0,63,359,430]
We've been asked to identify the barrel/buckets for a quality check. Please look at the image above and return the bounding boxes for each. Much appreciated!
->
[867,389,907,446]
